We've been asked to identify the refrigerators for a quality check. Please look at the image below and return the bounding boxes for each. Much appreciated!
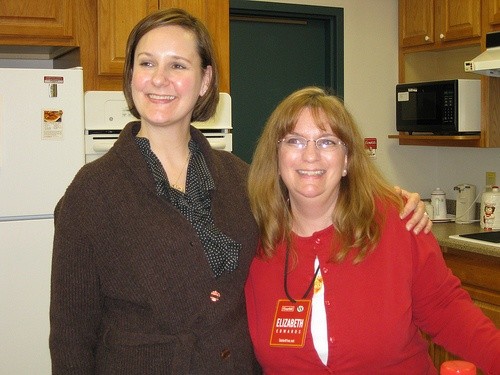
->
[0,67,85,375]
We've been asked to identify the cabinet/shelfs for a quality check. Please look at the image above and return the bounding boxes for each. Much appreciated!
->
[388,0,500,148]
[53,0,229,94]
[0,0,80,60]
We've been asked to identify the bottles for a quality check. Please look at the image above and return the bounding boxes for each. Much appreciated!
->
[431,187,446,219]
[479,184,500,229]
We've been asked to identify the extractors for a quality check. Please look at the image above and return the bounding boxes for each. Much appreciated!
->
[464,31,500,78]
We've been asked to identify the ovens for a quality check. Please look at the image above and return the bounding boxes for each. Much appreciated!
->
[85,90,234,166]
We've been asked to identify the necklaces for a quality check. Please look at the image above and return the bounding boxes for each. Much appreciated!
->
[170,151,189,191]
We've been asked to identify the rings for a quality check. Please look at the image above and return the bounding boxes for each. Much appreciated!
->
[424,212,430,219]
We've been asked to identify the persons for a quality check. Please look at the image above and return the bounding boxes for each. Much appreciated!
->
[48,7,432,375]
[244,87,499,375]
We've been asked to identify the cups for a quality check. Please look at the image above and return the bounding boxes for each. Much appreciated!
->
[422,201,434,220]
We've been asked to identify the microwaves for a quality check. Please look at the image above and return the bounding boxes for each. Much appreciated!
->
[395,79,481,135]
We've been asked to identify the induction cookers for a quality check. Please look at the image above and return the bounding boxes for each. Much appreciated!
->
[460,231,500,244]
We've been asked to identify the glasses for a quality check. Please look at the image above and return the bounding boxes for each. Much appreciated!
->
[278,137,346,151]
